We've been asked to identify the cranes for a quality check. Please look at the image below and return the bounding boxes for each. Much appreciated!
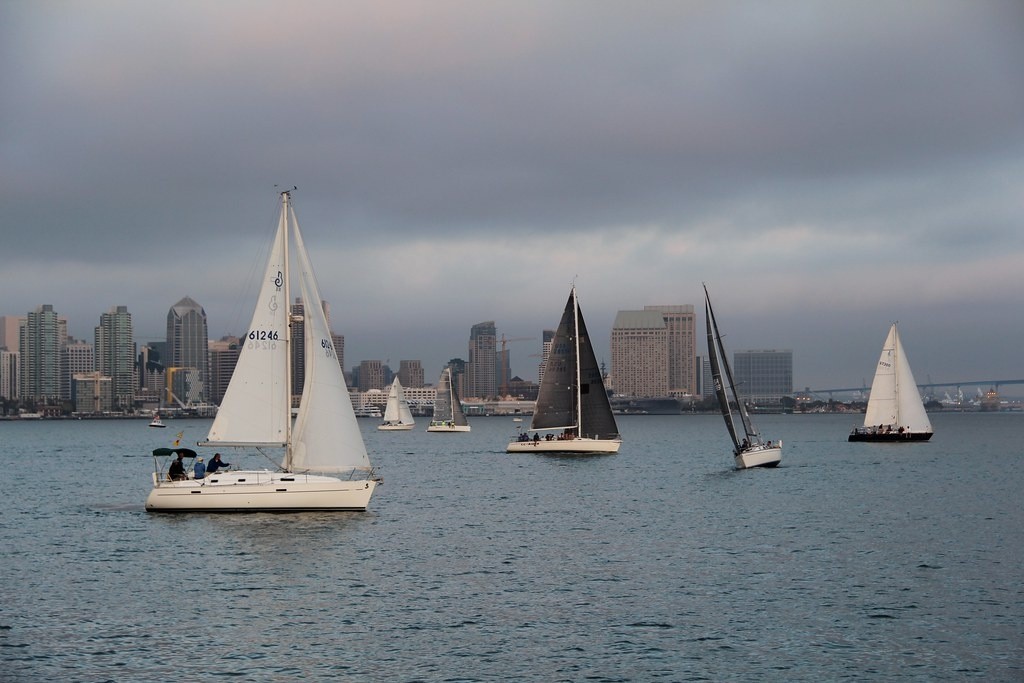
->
[488,332,539,397]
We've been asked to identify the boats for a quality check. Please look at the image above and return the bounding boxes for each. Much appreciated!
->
[147,415,167,428]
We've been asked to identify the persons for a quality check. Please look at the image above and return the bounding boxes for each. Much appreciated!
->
[767,441,773,449]
[742,438,748,447]
[169,451,186,481]
[854,423,911,434]
[517,433,529,441]
[194,457,206,479]
[206,453,232,473]
[533,432,540,441]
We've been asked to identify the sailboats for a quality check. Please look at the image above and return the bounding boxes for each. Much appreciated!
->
[702,281,783,470]
[848,321,936,442]
[425,367,471,432]
[376,375,416,430]
[143,182,386,513]
[505,273,624,455]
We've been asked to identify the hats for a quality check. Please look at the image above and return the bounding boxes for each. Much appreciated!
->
[173,459,179,463]
